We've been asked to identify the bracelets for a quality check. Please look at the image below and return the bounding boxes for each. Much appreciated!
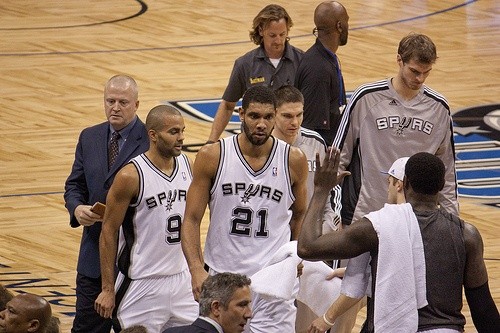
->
[322,313,336,326]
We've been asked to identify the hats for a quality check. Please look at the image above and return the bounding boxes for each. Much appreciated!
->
[379,156,410,181]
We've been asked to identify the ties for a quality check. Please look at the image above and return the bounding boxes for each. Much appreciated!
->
[108,132,121,171]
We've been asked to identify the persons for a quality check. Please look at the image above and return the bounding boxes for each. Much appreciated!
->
[64,75,151,333]
[160,271,253,333]
[0,284,61,333]
[181,1,500,333]
[94,105,205,333]
